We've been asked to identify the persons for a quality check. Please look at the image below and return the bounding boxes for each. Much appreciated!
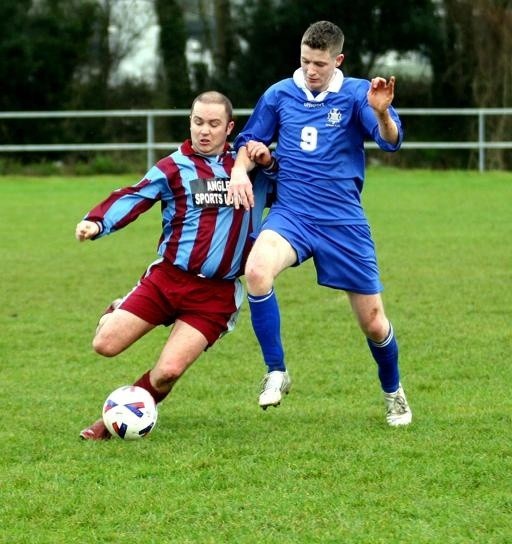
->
[75,90,279,441]
[225,20,413,428]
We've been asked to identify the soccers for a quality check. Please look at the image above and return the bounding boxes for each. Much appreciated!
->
[102,385,158,440]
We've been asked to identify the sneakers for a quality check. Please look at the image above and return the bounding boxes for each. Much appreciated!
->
[381,380,413,428]
[97,297,124,328]
[78,419,112,443]
[258,366,292,410]
